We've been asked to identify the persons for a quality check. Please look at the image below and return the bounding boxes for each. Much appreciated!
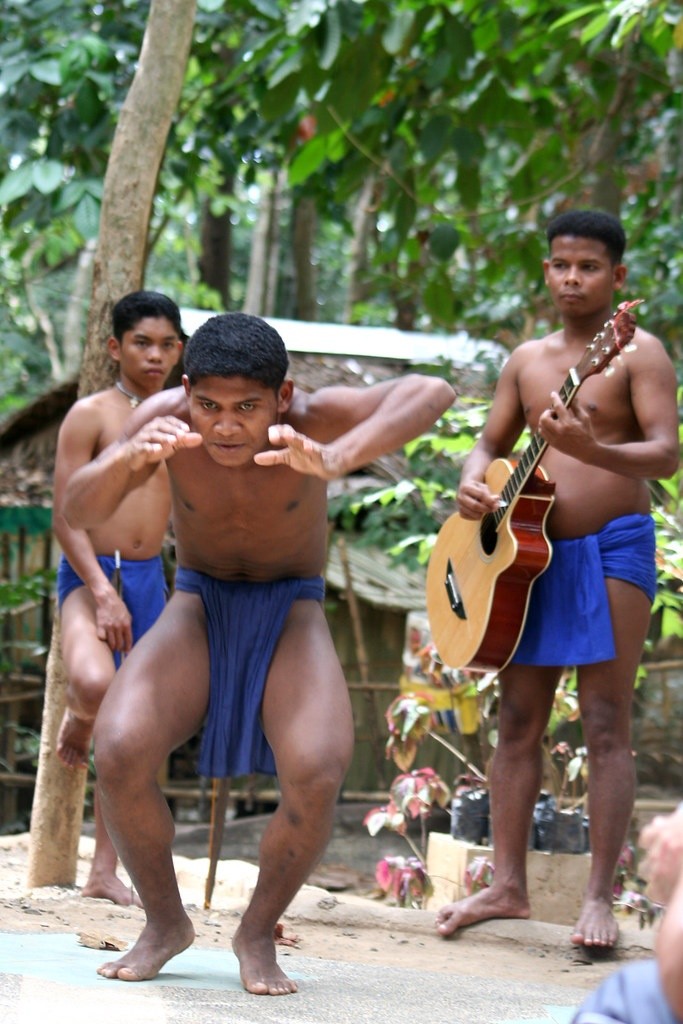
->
[60,312,458,997]
[571,801,683,1024]
[432,210,681,945]
[48,292,183,910]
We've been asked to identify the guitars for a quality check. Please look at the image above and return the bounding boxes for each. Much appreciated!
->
[423,298,645,677]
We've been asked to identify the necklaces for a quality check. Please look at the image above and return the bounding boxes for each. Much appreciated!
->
[116,381,144,409]
